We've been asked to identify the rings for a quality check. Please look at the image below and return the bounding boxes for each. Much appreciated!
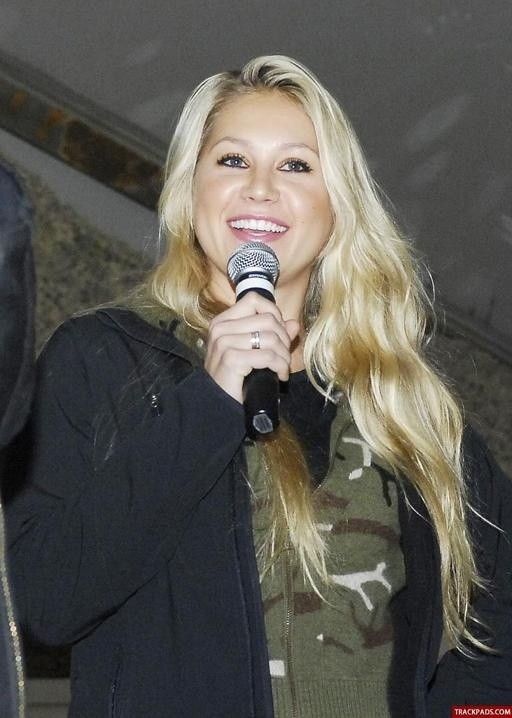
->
[248,330,262,350]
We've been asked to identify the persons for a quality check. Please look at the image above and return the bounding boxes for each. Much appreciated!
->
[0,52,510,717]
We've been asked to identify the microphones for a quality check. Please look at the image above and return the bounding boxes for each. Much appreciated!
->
[226,243,281,440]
